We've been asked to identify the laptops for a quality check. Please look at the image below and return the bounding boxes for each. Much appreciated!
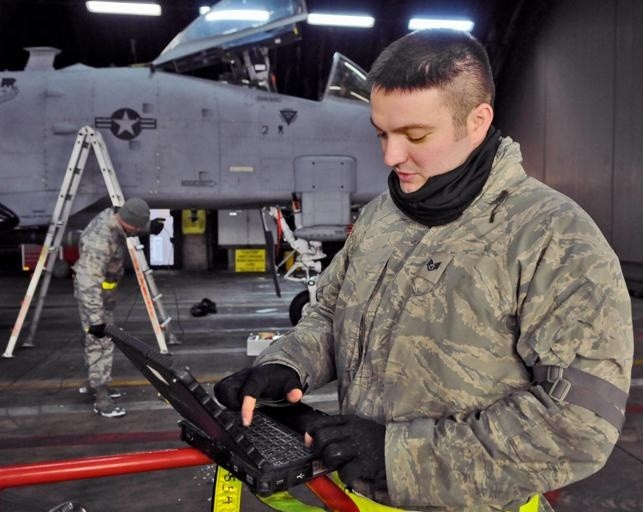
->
[103,324,332,498]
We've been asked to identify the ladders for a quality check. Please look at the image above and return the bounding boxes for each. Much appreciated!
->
[1,123,184,358]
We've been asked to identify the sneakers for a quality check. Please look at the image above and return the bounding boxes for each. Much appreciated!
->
[89,388,127,399]
[93,396,126,417]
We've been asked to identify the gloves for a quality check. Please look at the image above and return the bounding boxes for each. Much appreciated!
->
[306,415,385,487]
[88,323,105,338]
[213,363,302,412]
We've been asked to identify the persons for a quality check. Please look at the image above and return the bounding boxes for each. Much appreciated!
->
[215,27,634,512]
[74,198,149,420]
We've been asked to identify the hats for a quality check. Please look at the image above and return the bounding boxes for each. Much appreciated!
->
[118,197,150,227]
